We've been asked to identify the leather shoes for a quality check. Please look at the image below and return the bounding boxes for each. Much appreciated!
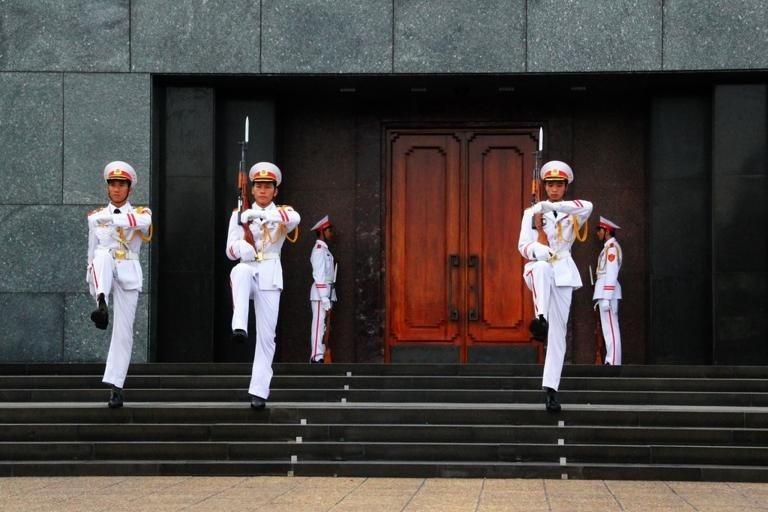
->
[230,331,249,344]
[545,391,561,411]
[250,396,265,410]
[529,319,545,342]
[110,389,123,408]
[91,309,108,329]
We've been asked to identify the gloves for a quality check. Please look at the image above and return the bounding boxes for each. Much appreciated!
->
[321,298,330,311]
[86,270,95,297]
[239,241,257,261]
[240,209,261,224]
[96,216,112,224]
[601,300,611,312]
[531,201,556,216]
[534,245,555,262]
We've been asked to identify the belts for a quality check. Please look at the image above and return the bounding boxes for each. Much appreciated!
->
[555,251,571,259]
[240,252,280,263]
[326,275,334,281]
[111,249,140,260]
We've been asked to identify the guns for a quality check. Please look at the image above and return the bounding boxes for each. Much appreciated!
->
[587,266,604,363]
[237,116,257,257]
[322,263,338,364]
[530,127,549,248]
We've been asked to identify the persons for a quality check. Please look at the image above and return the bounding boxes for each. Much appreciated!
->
[309,214,338,367]
[224,161,302,408]
[596,215,623,367]
[86,160,153,408]
[517,160,594,412]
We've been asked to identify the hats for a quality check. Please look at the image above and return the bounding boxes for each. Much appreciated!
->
[248,162,283,187]
[103,161,137,191]
[540,160,575,185]
[309,215,333,233]
[596,216,622,232]
